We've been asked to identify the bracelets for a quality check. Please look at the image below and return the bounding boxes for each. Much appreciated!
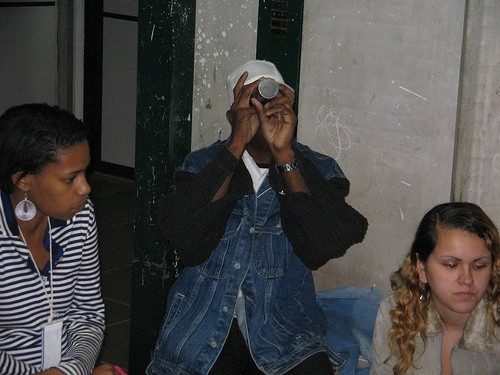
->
[275,161,300,176]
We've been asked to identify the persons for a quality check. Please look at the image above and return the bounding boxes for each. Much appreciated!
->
[371,200,500,375]
[145,58,368,375]
[0,103,127,375]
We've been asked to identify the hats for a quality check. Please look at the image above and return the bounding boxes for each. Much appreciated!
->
[225,59,295,108]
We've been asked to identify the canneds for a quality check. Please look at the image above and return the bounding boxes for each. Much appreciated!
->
[250,78,279,105]
